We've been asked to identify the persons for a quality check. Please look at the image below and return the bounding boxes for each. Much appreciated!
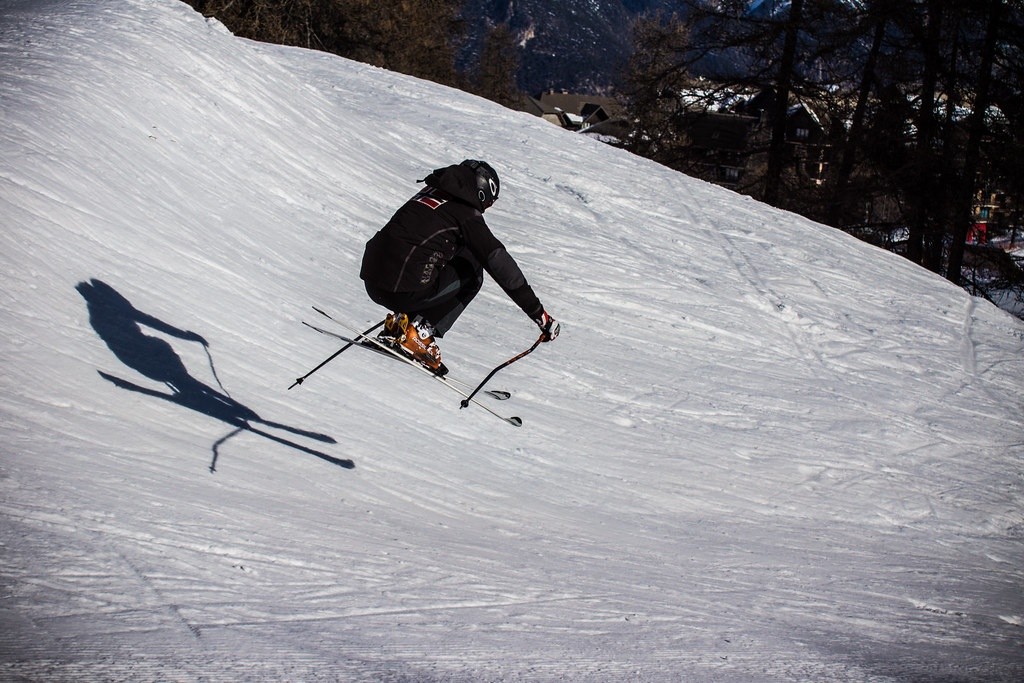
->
[359,159,560,376]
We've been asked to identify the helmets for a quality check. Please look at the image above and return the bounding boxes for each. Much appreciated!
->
[456,159,499,209]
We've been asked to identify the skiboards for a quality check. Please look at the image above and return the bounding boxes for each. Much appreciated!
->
[301,304,523,429]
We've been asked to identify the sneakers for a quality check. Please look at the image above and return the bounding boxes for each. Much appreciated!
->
[378,313,408,348]
[393,315,441,370]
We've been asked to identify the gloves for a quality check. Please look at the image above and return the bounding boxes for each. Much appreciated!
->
[531,304,560,341]
